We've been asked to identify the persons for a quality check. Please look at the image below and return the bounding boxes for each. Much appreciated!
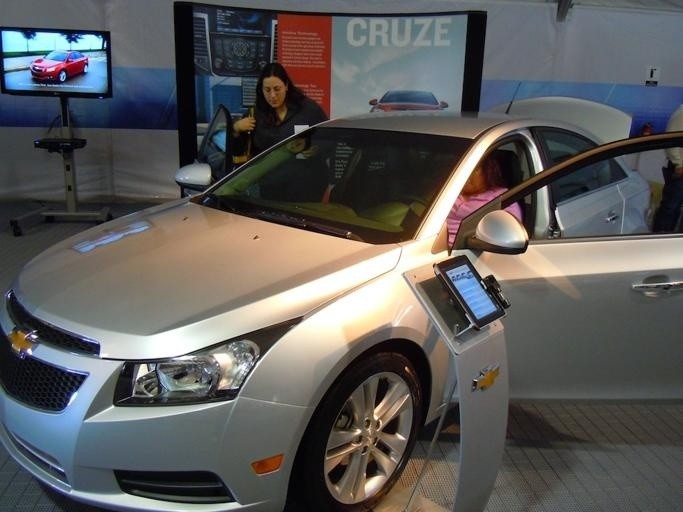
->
[230,59,332,206]
[411,148,525,251]
[652,103,682,233]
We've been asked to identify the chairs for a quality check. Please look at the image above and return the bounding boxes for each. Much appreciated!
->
[490,150,583,221]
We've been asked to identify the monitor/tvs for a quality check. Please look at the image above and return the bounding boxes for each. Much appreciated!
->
[0,26,113,99]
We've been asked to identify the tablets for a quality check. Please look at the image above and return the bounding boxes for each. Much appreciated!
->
[433,254,508,330]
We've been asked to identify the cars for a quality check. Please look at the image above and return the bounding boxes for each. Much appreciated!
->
[28,49,89,83]
[368,88,449,116]
[0,79,683,512]
